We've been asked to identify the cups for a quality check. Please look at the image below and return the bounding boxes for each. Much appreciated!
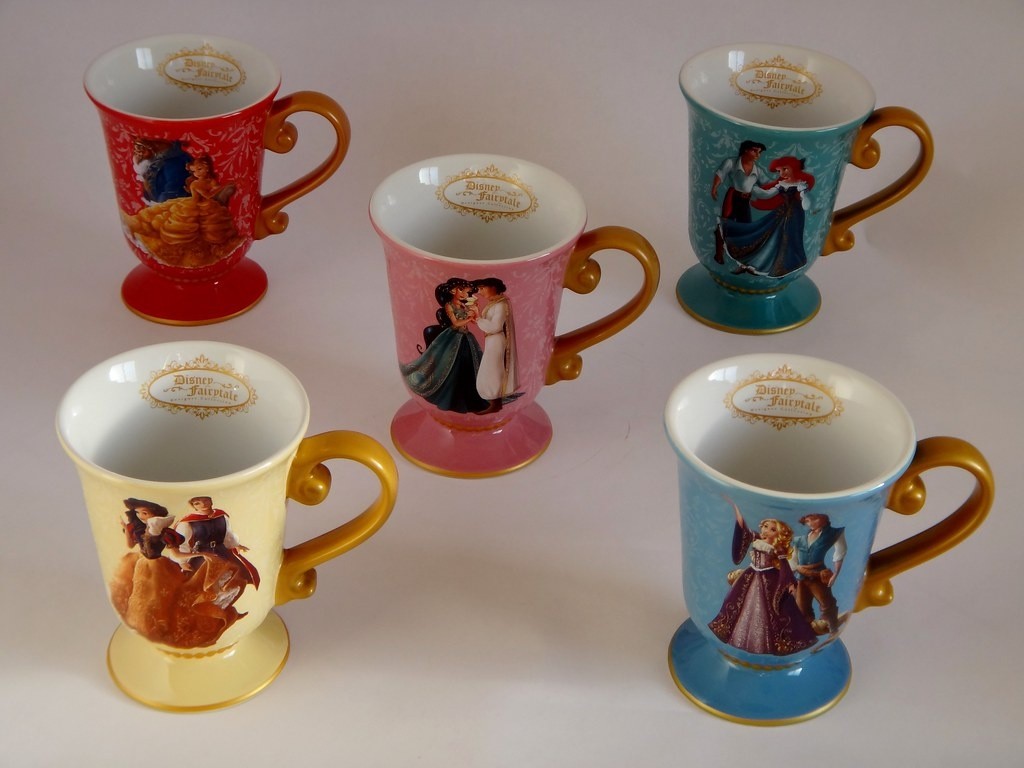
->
[677,42,935,335]
[662,354,994,726]
[369,154,662,479]
[55,341,398,713]
[84,34,350,326]
